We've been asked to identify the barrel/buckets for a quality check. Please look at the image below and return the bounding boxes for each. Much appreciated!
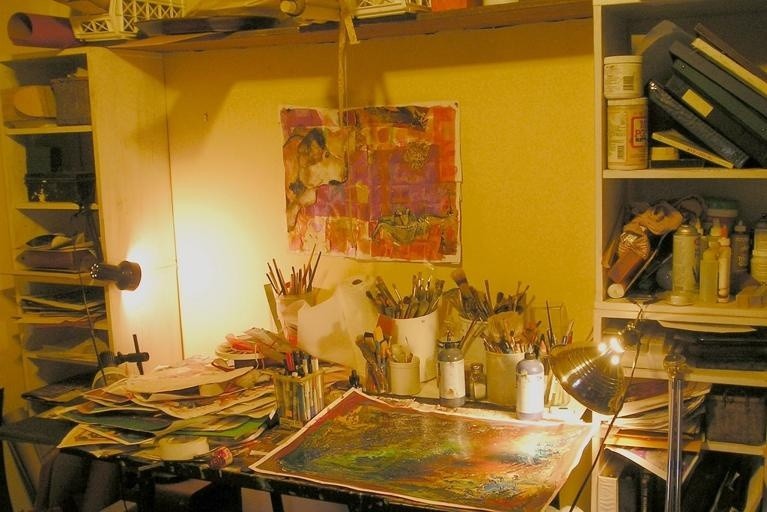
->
[388,309,441,382]
[458,317,489,376]
[486,351,532,405]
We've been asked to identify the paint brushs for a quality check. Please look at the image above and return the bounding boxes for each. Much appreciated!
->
[355,326,414,395]
[456,301,595,354]
[266,243,322,296]
[365,270,530,321]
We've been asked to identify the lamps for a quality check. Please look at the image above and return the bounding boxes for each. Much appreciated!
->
[548,317,693,512]
[89,260,149,370]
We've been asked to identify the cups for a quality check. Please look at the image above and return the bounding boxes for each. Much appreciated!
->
[274,291,313,343]
[389,355,422,396]
[486,351,526,407]
[364,361,388,394]
[377,306,440,384]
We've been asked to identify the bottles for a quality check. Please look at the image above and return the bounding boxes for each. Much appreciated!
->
[207,446,234,468]
[469,362,487,401]
[515,352,545,421]
[437,342,466,407]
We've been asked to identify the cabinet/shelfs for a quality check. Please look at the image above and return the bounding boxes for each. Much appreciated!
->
[589,0,767,512]
[0,48,185,411]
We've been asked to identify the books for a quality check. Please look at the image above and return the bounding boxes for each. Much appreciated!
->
[647,21,766,170]
[607,379,712,442]
[596,452,765,512]
[10,231,103,320]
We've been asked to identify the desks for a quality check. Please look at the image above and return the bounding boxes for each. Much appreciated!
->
[0,367,589,512]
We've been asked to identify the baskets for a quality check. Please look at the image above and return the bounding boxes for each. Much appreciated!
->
[133,18,209,35]
[69,2,433,42]
[354,0,432,22]
[209,15,282,33]
[70,2,186,41]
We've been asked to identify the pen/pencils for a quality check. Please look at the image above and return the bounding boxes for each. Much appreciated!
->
[283,349,323,423]
